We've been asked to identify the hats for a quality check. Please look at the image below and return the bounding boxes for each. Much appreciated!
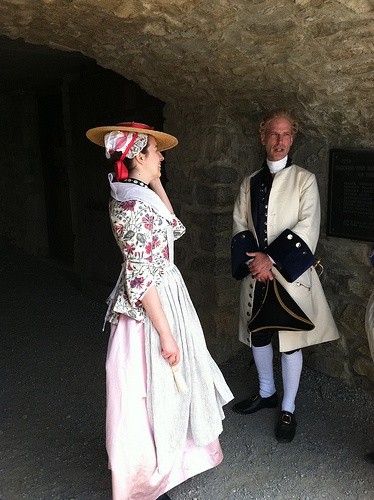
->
[85,123,178,153]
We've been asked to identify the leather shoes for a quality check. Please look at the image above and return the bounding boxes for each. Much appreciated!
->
[277,404,298,443]
[233,390,278,413]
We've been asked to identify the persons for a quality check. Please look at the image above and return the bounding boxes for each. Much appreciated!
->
[83,121,236,500]
[230,109,340,445]
